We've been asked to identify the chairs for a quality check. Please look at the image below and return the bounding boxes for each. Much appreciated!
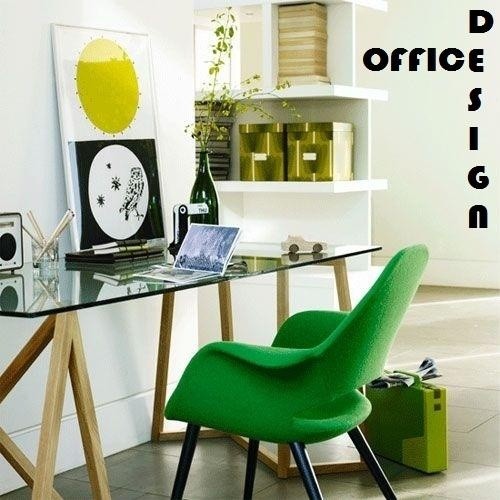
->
[161,244,429,499]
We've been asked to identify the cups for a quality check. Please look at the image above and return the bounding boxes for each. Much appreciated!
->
[33,272,61,306]
[30,237,61,277]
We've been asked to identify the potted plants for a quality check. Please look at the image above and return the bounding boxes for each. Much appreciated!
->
[183,5,301,225]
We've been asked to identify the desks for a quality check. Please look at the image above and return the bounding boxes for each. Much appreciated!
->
[0,240,381,499]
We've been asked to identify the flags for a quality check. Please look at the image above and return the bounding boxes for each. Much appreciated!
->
[281,234,328,254]
[281,255,327,265]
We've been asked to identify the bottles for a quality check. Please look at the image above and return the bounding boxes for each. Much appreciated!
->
[189,151,219,226]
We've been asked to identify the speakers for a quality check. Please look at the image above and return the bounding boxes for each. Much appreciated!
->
[0,212,24,276]
[0,274,25,312]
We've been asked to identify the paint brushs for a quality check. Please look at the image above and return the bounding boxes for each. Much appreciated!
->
[22,208,74,262]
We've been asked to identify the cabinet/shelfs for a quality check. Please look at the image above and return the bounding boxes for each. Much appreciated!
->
[195,1,390,349]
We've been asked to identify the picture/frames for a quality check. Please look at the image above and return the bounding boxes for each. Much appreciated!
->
[48,22,169,255]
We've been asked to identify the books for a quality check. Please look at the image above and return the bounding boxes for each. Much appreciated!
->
[130,222,243,284]
[92,271,137,286]
[65,238,165,262]
